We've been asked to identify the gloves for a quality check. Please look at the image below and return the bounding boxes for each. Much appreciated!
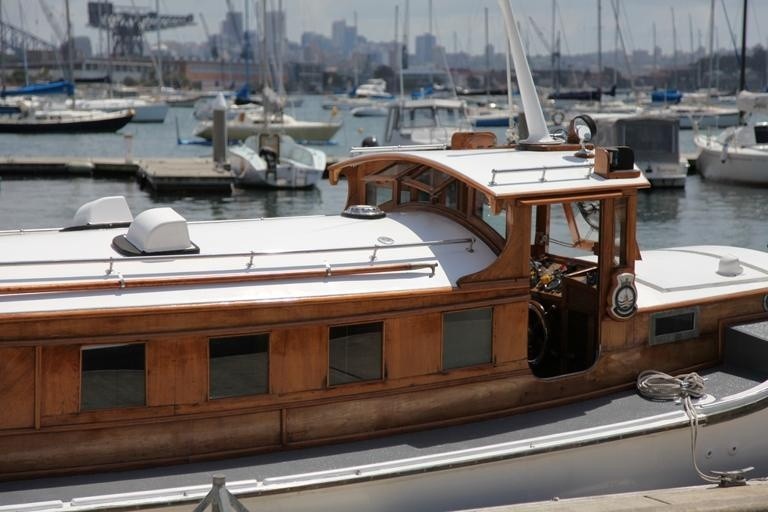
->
[227,132,327,190]
[0,1,768,511]
[575,112,690,189]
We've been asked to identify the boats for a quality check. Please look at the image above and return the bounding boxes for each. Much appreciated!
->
[227,132,327,190]
[575,112,690,189]
[0,1,768,511]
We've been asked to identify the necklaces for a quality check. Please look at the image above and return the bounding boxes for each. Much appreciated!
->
[694,0,768,186]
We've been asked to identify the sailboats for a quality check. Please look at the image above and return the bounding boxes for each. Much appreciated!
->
[694,0,768,186]
[1,1,767,146]
